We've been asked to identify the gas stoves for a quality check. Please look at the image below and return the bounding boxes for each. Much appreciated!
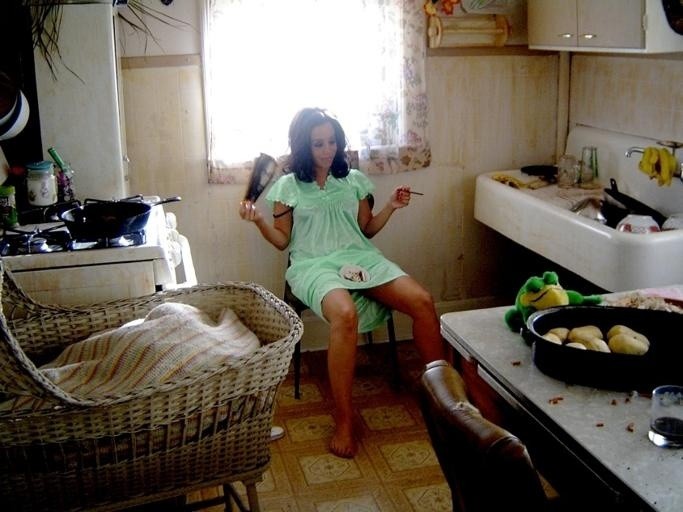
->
[0,193,146,256]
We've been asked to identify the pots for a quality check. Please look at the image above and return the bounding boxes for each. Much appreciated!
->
[569,178,668,232]
[60,196,183,243]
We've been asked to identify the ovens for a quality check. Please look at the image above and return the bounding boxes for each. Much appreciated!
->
[11,212,199,307]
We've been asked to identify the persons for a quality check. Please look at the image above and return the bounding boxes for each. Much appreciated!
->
[240,109,447,457]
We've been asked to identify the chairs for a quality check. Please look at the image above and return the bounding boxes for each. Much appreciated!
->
[282,193,400,400]
[413,359,575,512]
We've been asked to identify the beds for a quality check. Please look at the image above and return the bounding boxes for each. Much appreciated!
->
[0,256,304,512]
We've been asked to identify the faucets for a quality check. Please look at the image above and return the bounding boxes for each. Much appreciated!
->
[625,146,683,182]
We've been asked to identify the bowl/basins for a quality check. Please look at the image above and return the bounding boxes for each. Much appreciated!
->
[616,214,661,233]
[662,214,683,229]
[519,304,683,396]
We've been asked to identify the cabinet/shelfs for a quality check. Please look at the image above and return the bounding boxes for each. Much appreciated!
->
[526,0,682,54]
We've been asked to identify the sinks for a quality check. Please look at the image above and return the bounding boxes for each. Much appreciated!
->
[474,126,683,294]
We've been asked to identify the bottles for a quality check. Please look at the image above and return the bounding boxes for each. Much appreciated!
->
[241,152,277,205]
[25,160,57,206]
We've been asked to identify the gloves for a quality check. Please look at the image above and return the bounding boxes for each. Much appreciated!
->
[640,146,676,187]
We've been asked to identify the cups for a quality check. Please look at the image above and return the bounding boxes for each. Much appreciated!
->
[647,385,683,449]
